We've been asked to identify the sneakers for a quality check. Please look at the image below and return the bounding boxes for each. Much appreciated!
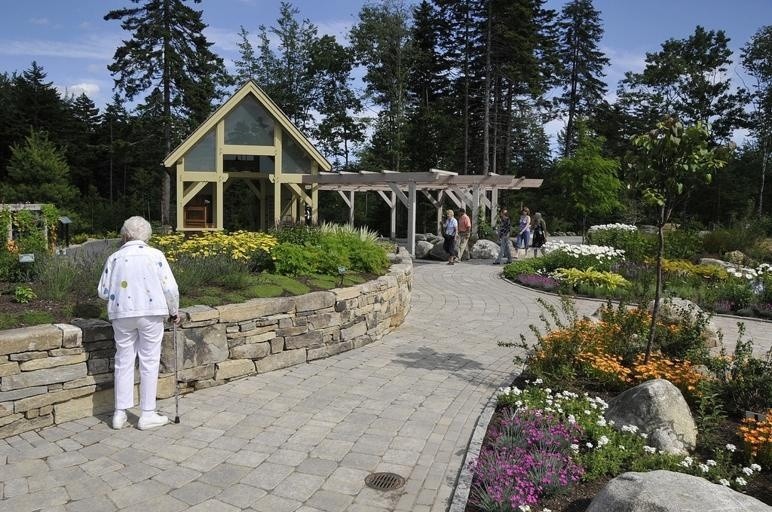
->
[112,411,127,431]
[138,412,169,430]
[493,255,518,265]
[447,257,460,265]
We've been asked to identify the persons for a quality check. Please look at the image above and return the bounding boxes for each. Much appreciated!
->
[529,212,548,258]
[492,207,512,264]
[454,208,472,262]
[97,214,181,431]
[440,209,458,265]
[512,206,531,259]
